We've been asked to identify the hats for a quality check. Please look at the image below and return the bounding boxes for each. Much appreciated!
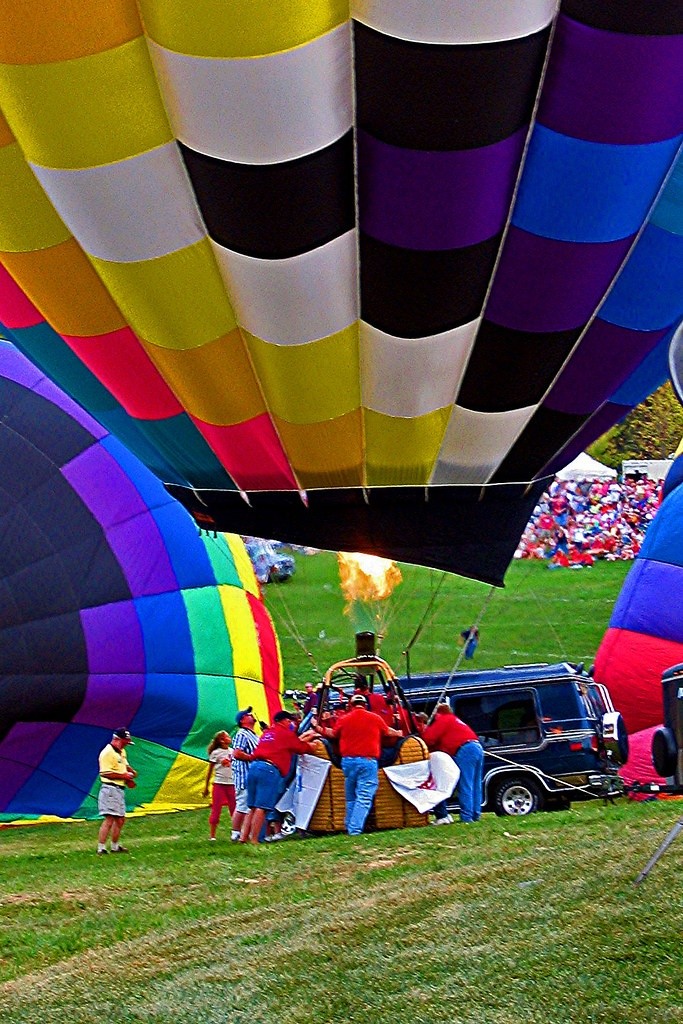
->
[350,695,367,704]
[113,727,134,745]
[274,710,294,722]
[235,706,253,726]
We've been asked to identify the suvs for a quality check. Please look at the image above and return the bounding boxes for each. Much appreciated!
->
[652,664,683,789]
[296,661,629,816]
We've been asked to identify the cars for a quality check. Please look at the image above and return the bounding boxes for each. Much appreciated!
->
[249,548,294,584]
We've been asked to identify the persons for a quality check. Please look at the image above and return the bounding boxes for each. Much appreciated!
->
[410,710,455,827]
[409,703,485,823]
[260,714,300,843]
[458,625,480,660]
[383,686,403,710]
[303,682,320,714]
[96,727,138,854]
[238,710,316,844]
[230,705,262,842]
[315,683,323,697]
[202,729,237,841]
[310,694,405,837]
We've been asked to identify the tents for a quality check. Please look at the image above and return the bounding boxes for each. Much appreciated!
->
[555,452,618,483]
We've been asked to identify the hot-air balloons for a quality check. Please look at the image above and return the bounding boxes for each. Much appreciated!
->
[0,0,683,832]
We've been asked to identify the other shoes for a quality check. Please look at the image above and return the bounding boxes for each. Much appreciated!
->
[96,849,109,854]
[264,836,272,841]
[272,833,285,840]
[231,829,240,842]
[111,846,128,853]
[433,814,454,826]
[208,836,216,842]
